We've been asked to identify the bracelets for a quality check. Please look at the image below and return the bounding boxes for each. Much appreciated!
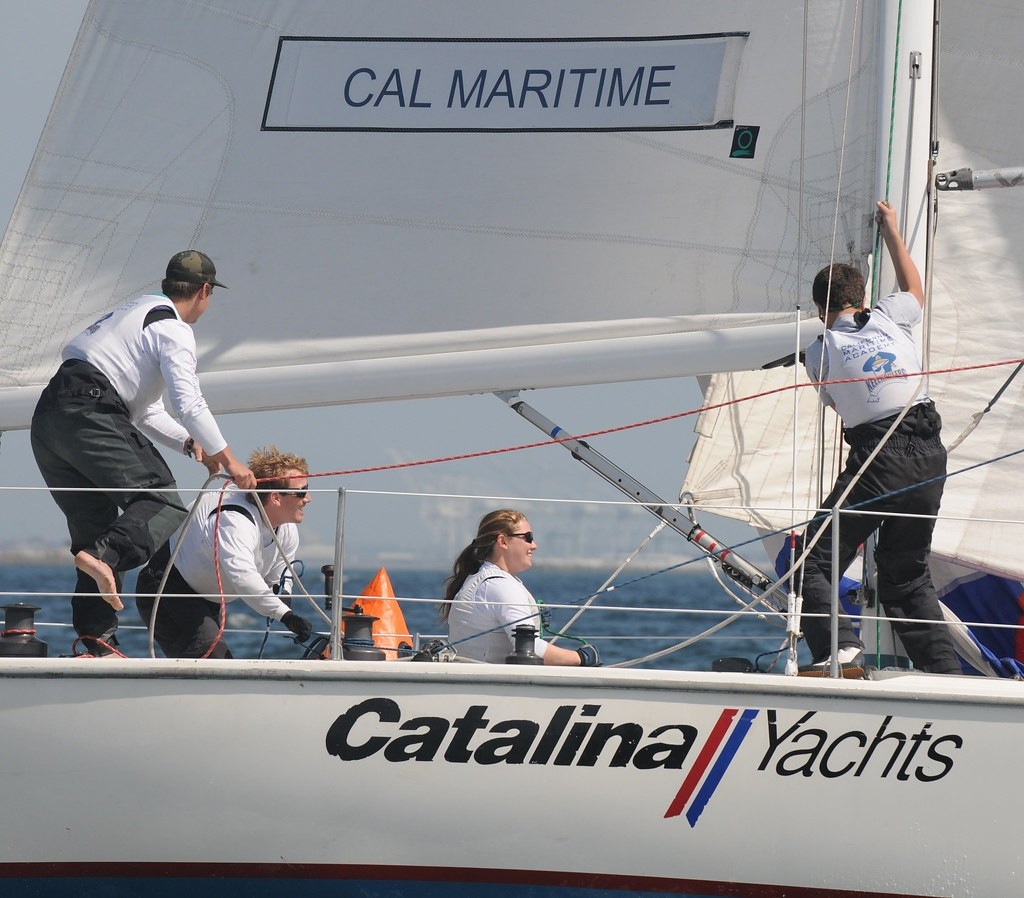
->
[186,439,194,458]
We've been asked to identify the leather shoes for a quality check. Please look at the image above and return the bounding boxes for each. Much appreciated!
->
[798,650,864,678]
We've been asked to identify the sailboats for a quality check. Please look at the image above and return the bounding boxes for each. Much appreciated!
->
[0,0,1024,898]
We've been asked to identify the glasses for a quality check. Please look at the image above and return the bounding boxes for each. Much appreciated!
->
[279,484,308,498]
[819,307,825,324]
[506,532,533,543]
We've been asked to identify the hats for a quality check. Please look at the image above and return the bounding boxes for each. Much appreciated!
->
[166,249,229,289]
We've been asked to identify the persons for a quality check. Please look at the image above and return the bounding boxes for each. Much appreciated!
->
[135,446,311,659]
[439,509,601,667]
[31,251,259,661]
[774,202,964,680]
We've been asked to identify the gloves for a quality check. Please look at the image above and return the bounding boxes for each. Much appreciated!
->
[575,644,603,666]
[279,589,292,609]
[281,611,312,644]
